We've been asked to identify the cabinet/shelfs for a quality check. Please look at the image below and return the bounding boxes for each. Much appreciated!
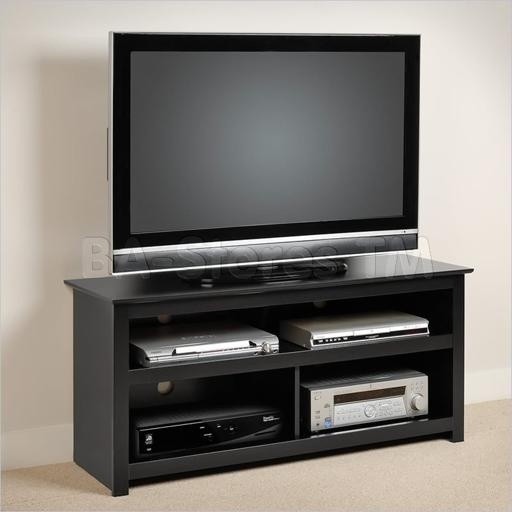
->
[62,253,476,498]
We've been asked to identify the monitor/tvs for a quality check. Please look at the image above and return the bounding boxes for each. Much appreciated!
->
[107,32,421,287]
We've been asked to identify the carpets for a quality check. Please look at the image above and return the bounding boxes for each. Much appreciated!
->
[0,399,512,511]
